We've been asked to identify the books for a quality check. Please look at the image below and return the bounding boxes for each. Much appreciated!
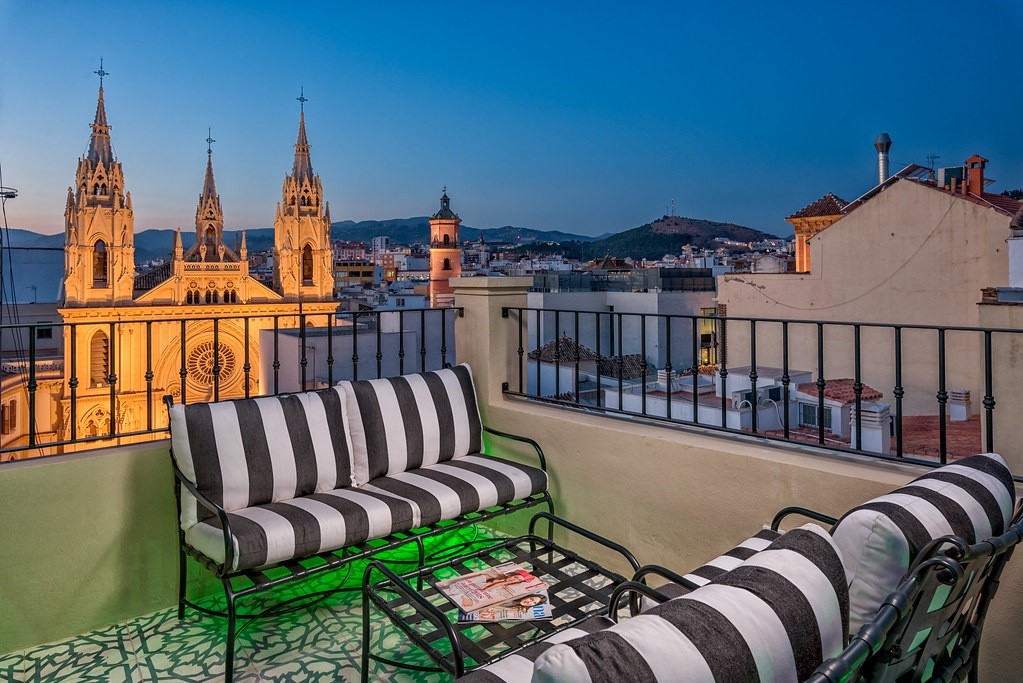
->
[435,561,553,625]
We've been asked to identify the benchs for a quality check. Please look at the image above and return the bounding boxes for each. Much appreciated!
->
[161,363,555,683]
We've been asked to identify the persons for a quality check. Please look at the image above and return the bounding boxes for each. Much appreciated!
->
[480,594,548,618]
[441,569,535,606]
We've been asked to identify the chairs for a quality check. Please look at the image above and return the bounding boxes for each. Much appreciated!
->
[454,454,1023,683]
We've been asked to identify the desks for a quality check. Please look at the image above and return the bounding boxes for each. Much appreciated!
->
[361,511,647,683]
